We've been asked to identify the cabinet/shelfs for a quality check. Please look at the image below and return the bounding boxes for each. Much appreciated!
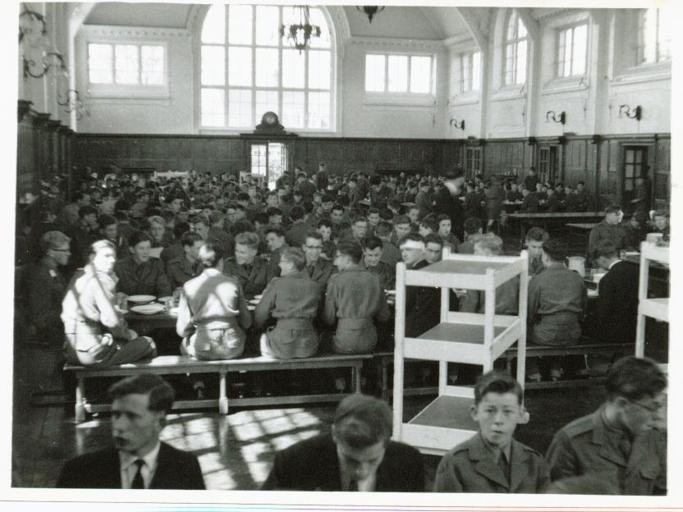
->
[632,239,673,381]
[390,243,524,449]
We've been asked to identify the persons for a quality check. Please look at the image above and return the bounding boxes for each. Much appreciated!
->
[360,237,396,307]
[255,248,322,388]
[316,164,487,241]
[652,210,669,243]
[596,239,641,343]
[262,228,293,284]
[588,204,631,264]
[472,232,519,316]
[283,166,316,245]
[523,227,549,278]
[233,170,283,230]
[114,231,172,301]
[56,166,114,239]
[114,168,233,242]
[176,241,252,398]
[54,373,206,489]
[15,230,72,351]
[300,232,338,289]
[630,177,649,212]
[58,238,157,365]
[165,232,204,287]
[399,232,459,388]
[433,369,549,494]
[529,238,585,383]
[625,212,649,243]
[544,355,667,495]
[496,164,595,211]
[324,238,390,393]
[259,392,426,492]
[222,231,267,297]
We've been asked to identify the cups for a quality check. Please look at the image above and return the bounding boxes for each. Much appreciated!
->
[590,268,597,280]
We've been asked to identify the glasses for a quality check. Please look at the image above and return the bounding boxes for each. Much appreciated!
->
[305,244,325,249]
[52,247,71,253]
[628,393,668,412]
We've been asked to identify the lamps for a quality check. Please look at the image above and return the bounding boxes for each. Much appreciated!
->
[545,110,565,129]
[355,6,385,23]
[278,4,321,55]
[18,12,48,50]
[56,91,90,124]
[24,52,70,86]
[449,119,464,132]
[618,102,642,122]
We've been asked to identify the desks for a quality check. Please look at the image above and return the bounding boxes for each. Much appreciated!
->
[562,221,598,234]
[505,209,610,241]
[122,297,273,326]
[385,267,609,308]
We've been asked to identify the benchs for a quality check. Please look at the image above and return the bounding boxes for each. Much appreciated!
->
[376,340,636,407]
[61,352,375,424]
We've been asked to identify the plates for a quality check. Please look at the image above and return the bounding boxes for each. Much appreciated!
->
[248,300,260,304]
[248,305,257,311]
[127,294,157,305]
[169,307,178,318]
[158,296,176,303]
[130,304,165,314]
[386,287,396,297]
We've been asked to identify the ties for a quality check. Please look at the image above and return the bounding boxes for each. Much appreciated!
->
[131,460,146,489]
[347,479,358,492]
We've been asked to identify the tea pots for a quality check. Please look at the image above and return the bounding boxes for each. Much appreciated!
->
[563,255,587,278]
